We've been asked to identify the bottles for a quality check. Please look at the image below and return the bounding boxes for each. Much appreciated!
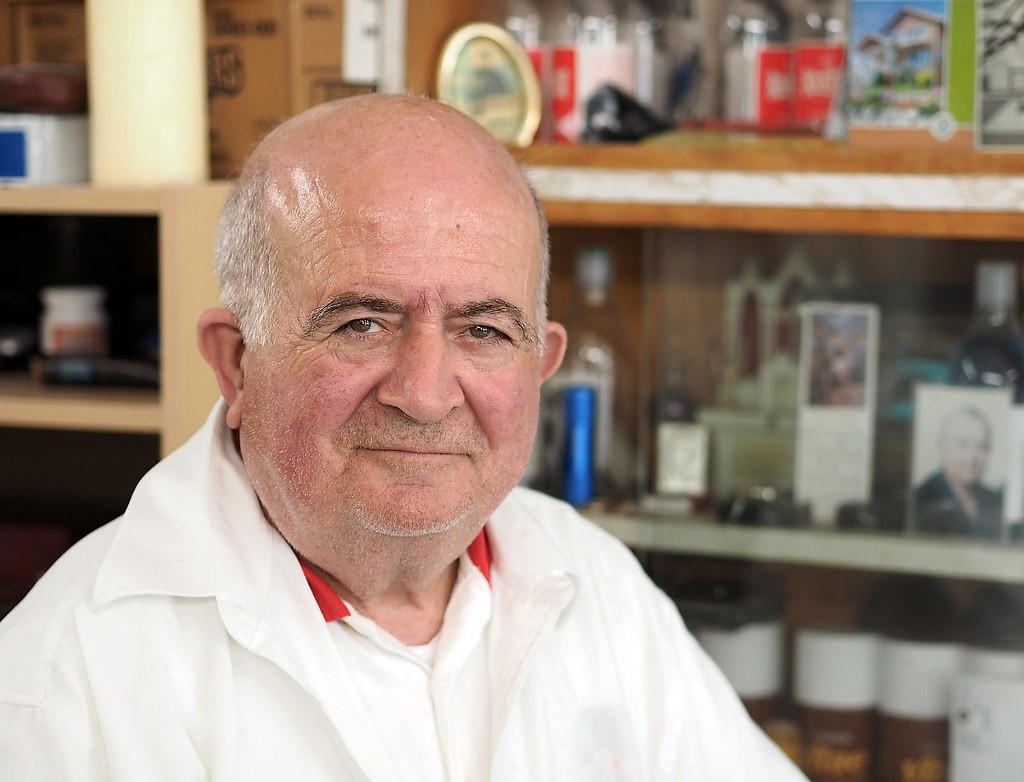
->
[949,259,1024,402]
[554,252,628,513]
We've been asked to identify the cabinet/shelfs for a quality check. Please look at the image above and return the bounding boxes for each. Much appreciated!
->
[0,179,235,460]
[379,0,1022,782]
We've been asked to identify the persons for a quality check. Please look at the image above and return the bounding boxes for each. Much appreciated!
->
[910,400,1001,541]
[0,95,806,782]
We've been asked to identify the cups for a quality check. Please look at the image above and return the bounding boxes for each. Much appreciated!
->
[38,288,109,359]
